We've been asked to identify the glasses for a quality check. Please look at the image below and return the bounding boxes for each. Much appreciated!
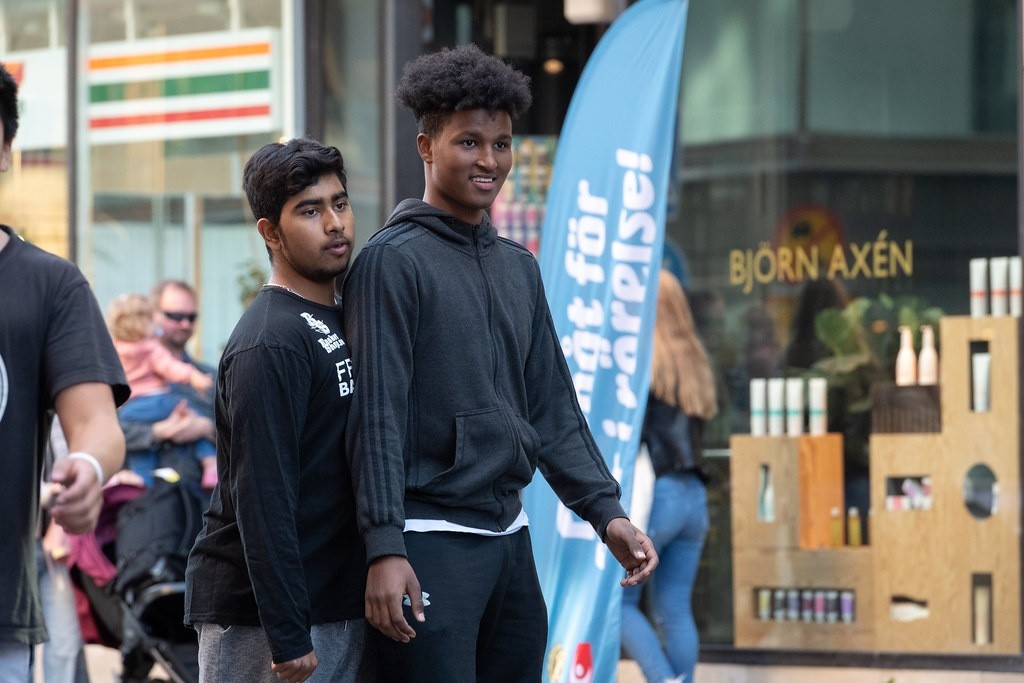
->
[160,309,196,324]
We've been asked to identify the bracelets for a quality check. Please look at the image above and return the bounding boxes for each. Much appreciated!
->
[66,452,103,486]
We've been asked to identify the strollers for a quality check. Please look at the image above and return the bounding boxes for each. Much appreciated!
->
[71,484,201,683]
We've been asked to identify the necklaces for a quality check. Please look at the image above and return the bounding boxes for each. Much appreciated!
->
[263,284,338,306]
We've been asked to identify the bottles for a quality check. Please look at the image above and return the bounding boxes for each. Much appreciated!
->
[829,508,842,546]
[846,507,862,547]
[895,326,917,387]
[917,324,939,387]
[757,589,854,625]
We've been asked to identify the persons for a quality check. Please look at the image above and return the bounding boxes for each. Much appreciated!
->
[0,63,132,683]
[183,138,367,683]
[35,278,218,683]
[621,266,716,683]
[687,270,871,547]
[342,42,658,683]
